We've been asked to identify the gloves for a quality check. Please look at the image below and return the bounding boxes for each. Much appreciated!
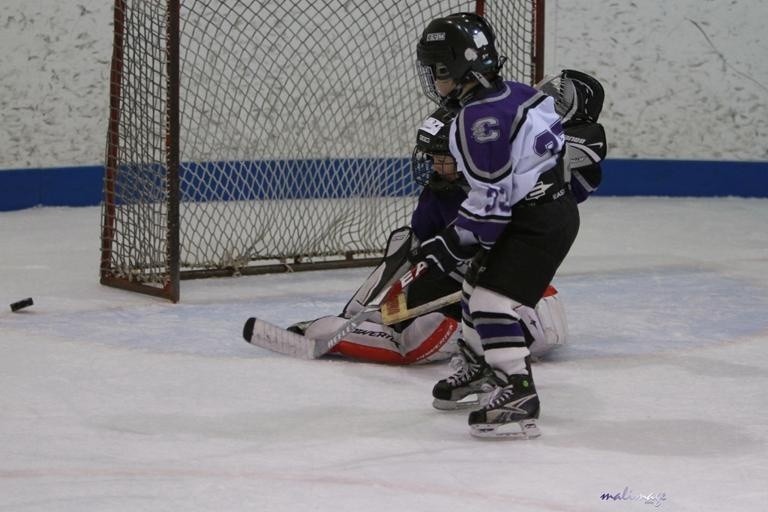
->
[417,225,480,279]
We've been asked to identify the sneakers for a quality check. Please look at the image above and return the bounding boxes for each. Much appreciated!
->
[286,320,314,335]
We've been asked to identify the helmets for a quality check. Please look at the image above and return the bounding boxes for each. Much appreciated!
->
[416,12,507,108]
[413,108,465,191]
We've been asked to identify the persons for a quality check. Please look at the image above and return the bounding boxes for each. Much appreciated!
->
[283,68,605,364]
[418,10,576,425]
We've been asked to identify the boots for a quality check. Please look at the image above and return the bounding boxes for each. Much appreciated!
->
[433,338,497,400]
[468,354,540,424]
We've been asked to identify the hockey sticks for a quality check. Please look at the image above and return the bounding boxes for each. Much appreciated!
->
[242,263,462,360]
